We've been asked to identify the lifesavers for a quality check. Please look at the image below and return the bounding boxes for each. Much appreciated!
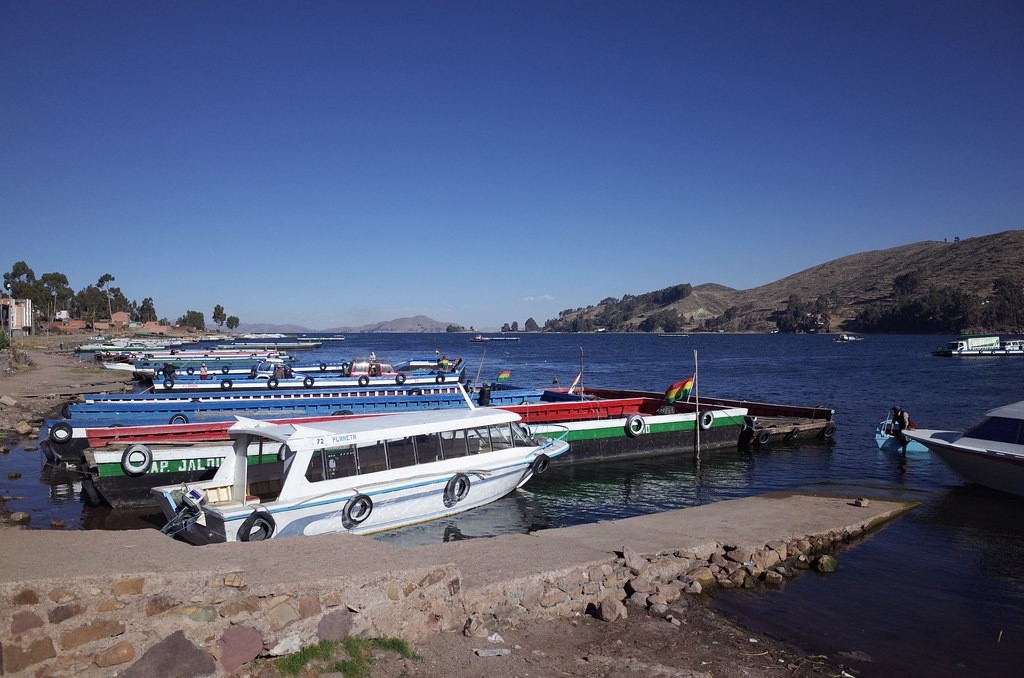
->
[49,422,73,443]
[531,453,551,475]
[699,409,715,431]
[153,361,448,404]
[512,422,531,441]
[120,443,154,478]
[625,413,646,438]
[102,341,303,362]
[342,492,374,525]
[445,472,471,503]
[235,510,277,543]
[277,443,286,462]
[978,349,1010,355]
[756,424,838,445]
[108,407,445,430]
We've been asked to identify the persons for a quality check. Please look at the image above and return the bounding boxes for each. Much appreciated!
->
[273,365,292,379]
[163,363,181,381]
[368,351,377,375]
[476,382,491,407]
[200,363,208,380]
[59,343,63,350]
[463,380,473,393]
[212,373,216,380]
[890,406,910,438]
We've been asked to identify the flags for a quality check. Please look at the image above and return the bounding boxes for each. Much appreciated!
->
[497,370,510,383]
[438,357,448,368]
[661,376,693,406]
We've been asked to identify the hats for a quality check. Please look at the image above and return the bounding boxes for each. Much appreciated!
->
[202,363,205,366]
[891,406,901,411]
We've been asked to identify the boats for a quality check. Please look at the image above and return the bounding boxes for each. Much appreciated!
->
[875,418,930,451]
[900,401,1024,496]
[831,334,865,344]
[39,334,835,545]
[937,335,1024,356]
